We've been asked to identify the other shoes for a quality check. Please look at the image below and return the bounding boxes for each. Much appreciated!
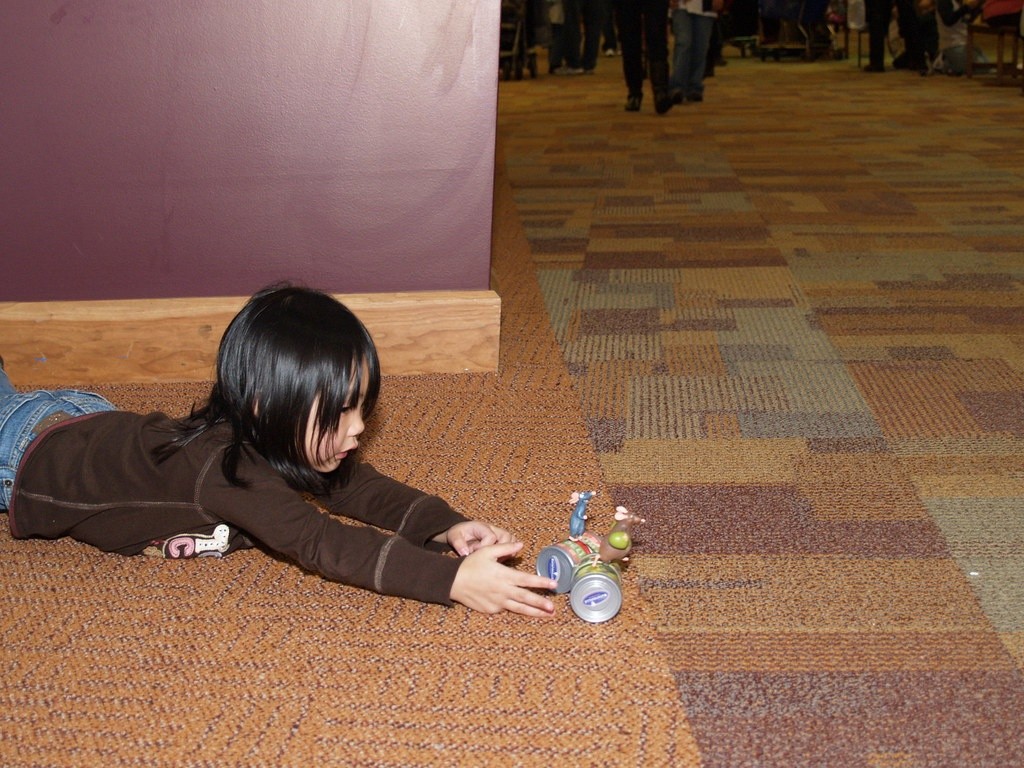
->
[553,65,594,76]
[625,94,642,112]
[655,88,681,115]
[605,49,614,57]
[863,64,884,73]
[686,87,702,103]
[617,49,622,56]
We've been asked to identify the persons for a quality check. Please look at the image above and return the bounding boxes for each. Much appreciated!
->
[0,278,558,616]
[501,0,728,116]
[864,0,980,77]
